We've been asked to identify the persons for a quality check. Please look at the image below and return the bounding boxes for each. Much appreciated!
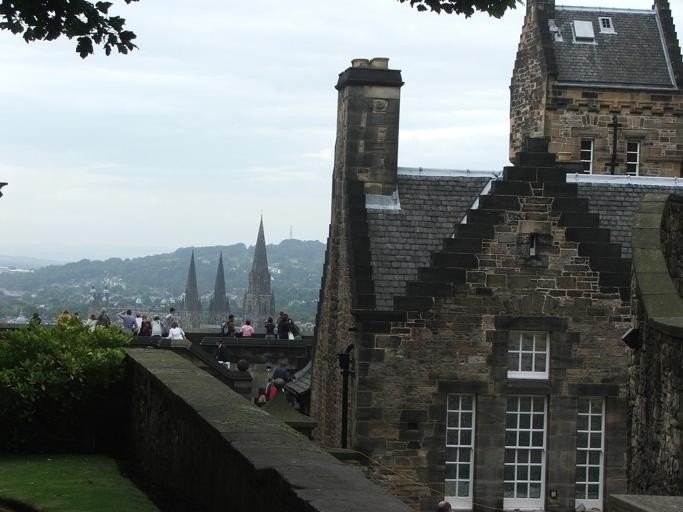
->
[277,311,303,340]
[226,314,236,337]
[59,310,71,319]
[31,312,41,324]
[239,320,254,337]
[84,314,98,333]
[117,310,152,336]
[165,306,177,336]
[151,315,167,337]
[71,310,82,319]
[221,321,228,337]
[97,307,111,328]
[264,316,276,338]
[257,359,293,406]
[167,321,186,338]
